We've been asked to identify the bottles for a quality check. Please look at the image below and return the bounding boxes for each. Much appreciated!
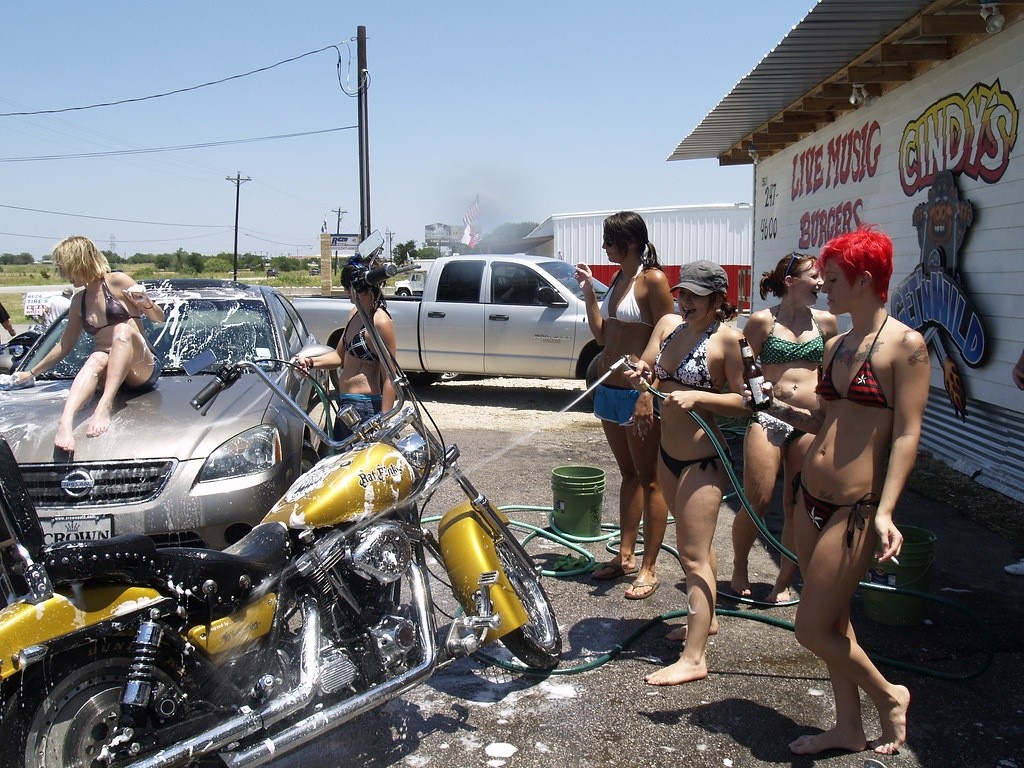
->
[738,338,770,411]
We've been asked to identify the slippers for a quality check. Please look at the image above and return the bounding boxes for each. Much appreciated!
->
[625,580,660,599]
[592,563,639,580]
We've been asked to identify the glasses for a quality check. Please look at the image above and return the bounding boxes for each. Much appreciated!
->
[786,251,807,274]
[602,235,621,246]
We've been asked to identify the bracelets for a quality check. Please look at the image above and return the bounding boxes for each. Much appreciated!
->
[30,370,36,376]
[144,303,154,310]
[306,356,314,370]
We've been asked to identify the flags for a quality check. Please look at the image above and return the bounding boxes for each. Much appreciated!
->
[461,195,482,249]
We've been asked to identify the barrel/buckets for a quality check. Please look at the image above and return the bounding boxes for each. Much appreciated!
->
[860,524,938,628]
[551,465,607,538]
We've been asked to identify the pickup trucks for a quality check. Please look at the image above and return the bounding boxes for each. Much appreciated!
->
[290,253,614,388]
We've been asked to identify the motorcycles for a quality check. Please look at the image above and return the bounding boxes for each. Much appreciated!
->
[0,227,562,768]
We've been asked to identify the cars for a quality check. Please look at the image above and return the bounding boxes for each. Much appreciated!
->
[267,268,279,276]
[309,267,320,277]
[228,270,237,274]
[0,277,336,584]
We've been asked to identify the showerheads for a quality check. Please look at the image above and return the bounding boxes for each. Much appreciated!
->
[608,350,642,379]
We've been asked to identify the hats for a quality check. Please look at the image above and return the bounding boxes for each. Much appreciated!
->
[670,260,730,296]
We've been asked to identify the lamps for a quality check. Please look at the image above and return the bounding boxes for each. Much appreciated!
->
[979,4,1007,33]
[849,83,878,109]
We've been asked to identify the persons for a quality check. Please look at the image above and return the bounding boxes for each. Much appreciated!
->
[573,211,675,599]
[621,260,752,686]
[10,236,165,452]
[732,251,838,602]
[295,256,396,456]
[45,287,73,326]
[0,303,16,336]
[742,231,931,755]
